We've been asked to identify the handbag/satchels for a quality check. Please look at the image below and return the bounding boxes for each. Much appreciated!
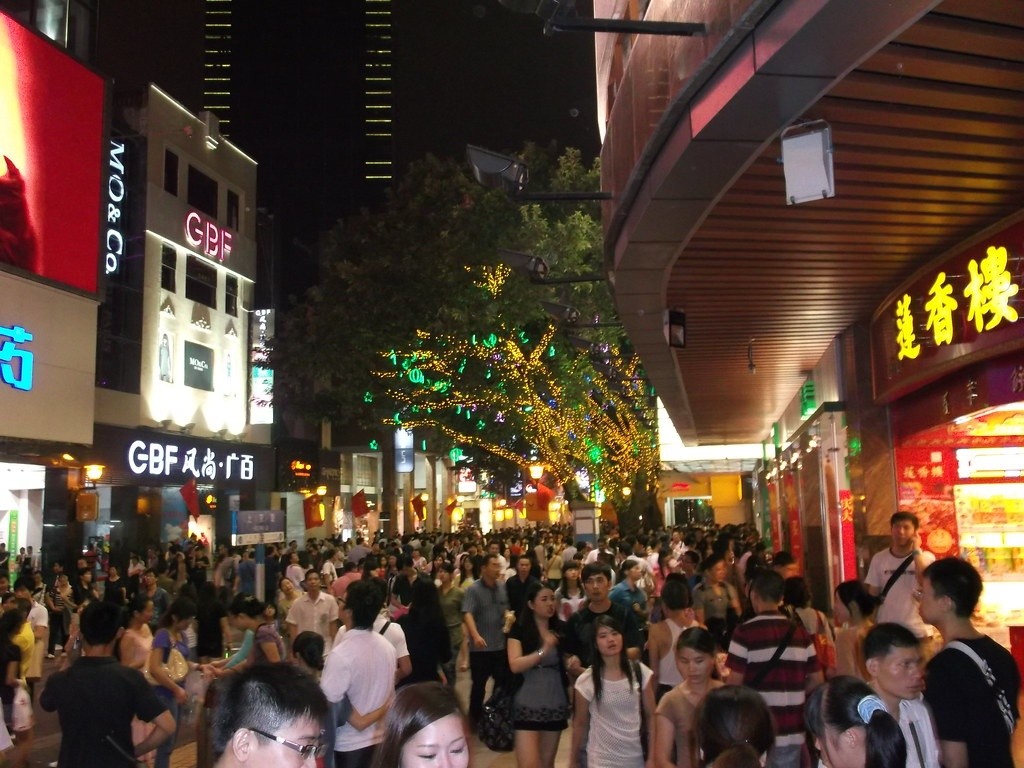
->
[12,679,36,731]
[636,562,656,591]
[477,689,515,752]
[143,631,189,686]
[635,660,648,765]
[544,571,548,584]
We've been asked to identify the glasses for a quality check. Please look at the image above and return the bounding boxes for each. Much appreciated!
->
[337,597,346,606]
[250,728,328,761]
[912,589,940,604]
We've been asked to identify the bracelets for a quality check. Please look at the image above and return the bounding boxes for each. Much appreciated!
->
[197,664,199,670]
[537,648,544,658]
[913,550,921,554]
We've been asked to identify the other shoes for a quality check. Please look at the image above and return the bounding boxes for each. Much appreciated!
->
[55,644,63,650]
[461,660,470,671]
[45,654,55,658]
[61,651,68,656]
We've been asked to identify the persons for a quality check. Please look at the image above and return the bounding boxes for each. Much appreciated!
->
[619,542,654,588]
[412,549,426,568]
[724,571,824,768]
[0,511,1024,768]
[507,582,580,768]
[543,547,564,589]
[285,568,339,664]
[233,552,256,596]
[647,581,707,701]
